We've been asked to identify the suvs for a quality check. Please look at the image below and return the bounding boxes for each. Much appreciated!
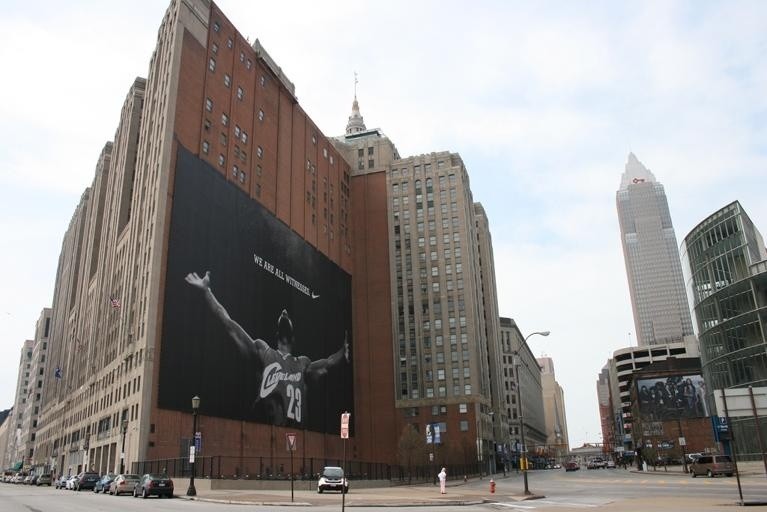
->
[688,452,736,478]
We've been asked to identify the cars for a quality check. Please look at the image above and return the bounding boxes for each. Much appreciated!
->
[316,466,349,493]
[133,473,173,498]
[0,471,141,496]
[544,450,712,472]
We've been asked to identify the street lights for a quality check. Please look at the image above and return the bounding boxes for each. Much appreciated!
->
[512,331,550,496]
[84,433,90,472]
[186,395,200,495]
[624,401,637,452]
[476,411,494,479]
[119,419,128,474]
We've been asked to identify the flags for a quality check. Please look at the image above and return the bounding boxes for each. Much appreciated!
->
[54,366,61,379]
[108,298,123,310]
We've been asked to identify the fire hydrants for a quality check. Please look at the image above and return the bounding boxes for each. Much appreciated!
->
[488,479,495,493]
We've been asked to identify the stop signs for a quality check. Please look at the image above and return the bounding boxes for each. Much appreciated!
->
[340,413,349,438]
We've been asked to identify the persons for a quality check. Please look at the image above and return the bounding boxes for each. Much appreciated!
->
[637,375,707,417]
[437,467,451,494]
[183,268,351,428]
